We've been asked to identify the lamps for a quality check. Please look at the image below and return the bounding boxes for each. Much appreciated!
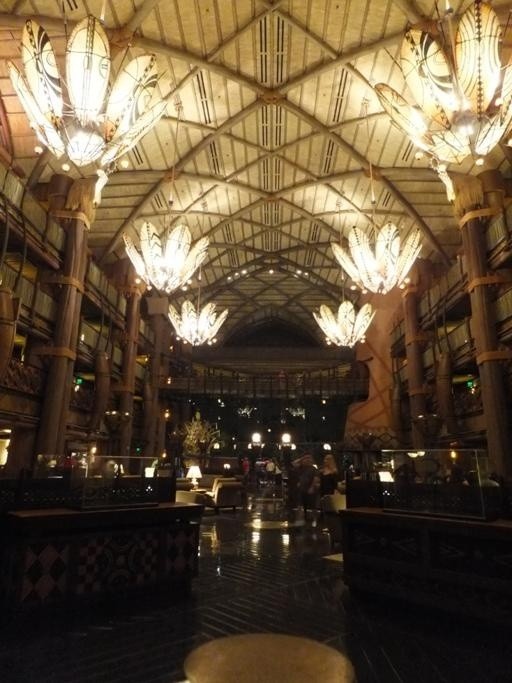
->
[313,202,377,348]
[167,202,229,347]
[330,101,423,296]
[5,0,167,171]
[373,1,510,167]
[121,107,209,294]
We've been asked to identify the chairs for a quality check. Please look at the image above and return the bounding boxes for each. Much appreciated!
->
[175,473,244,515]
[319,494,349,552]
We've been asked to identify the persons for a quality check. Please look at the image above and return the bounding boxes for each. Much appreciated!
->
[232,368,239,383]
[242,451,339,513]
[279,368,311,392]
[393,455,465,482]
